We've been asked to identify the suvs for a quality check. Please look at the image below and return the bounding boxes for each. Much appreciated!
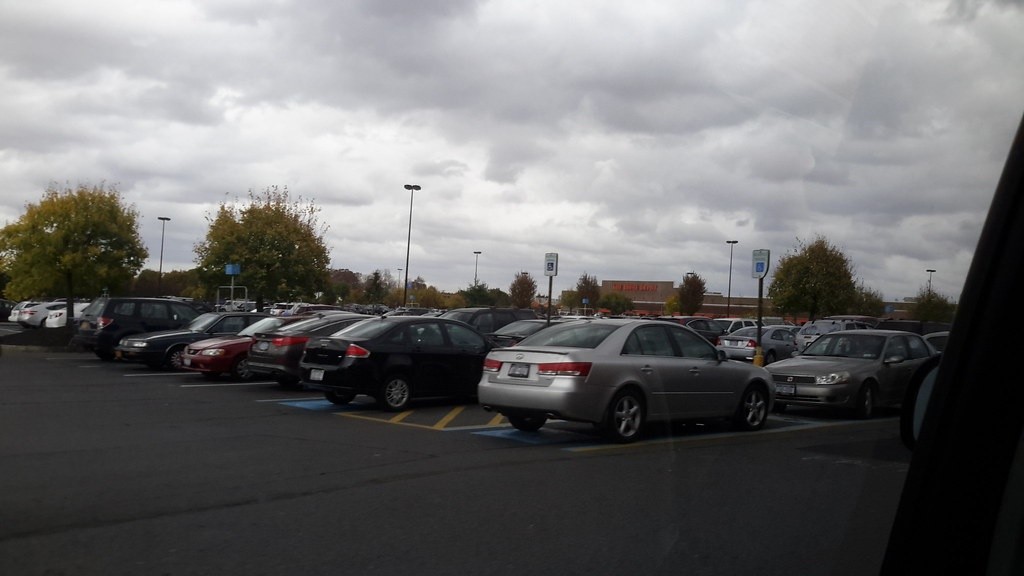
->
[72,296,201,361]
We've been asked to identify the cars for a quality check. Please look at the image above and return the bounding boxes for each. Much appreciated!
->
[181,315,309,381]
[248,310,375,389]
[764,330,940,416]
[660,316,885,365]
[7,299,90,328]
[217,297,659,347]
[115,313,269,370]
[298,314,500,411]
[477,318,775,442]
